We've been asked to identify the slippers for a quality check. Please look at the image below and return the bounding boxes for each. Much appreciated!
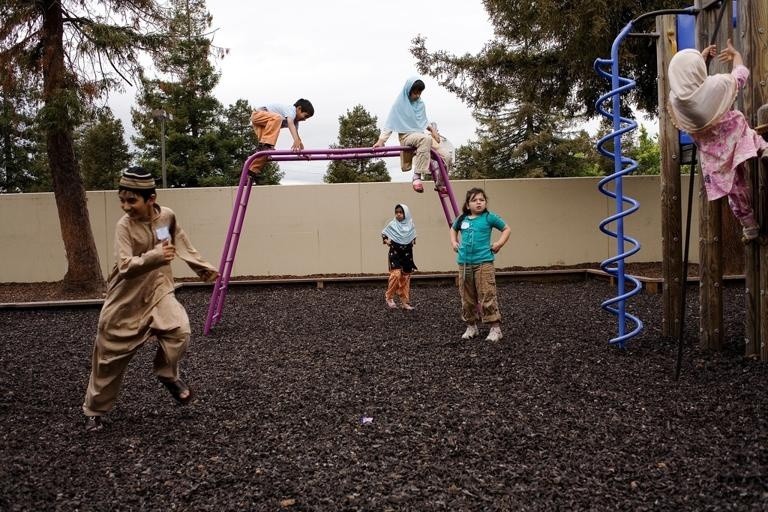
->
[157,376,193,403]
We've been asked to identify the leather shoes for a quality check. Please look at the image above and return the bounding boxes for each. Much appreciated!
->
[434,184,447,194]
[412,178,424,193]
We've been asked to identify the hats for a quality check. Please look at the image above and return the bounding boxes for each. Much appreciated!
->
[118,166,157,190]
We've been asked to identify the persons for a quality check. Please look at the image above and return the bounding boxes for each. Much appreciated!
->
[80,166,226,433]
[663,37,768,244]
[449,188,512,343]
[380,202,418,312]
[372,76,455,194]
[247,99,314,182]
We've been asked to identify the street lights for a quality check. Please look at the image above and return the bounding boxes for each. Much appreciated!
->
[153,110,175,189]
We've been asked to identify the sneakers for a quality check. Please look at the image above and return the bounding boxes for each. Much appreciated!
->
[760,148,768,166]
[742,225,760,240]
[402,305,415,310]
[84,415,104,432]
[255,143,276,153]
[386,299,397,309]
[247,169,265,180]
[462,325,480,340]
[485,327,504,343]
